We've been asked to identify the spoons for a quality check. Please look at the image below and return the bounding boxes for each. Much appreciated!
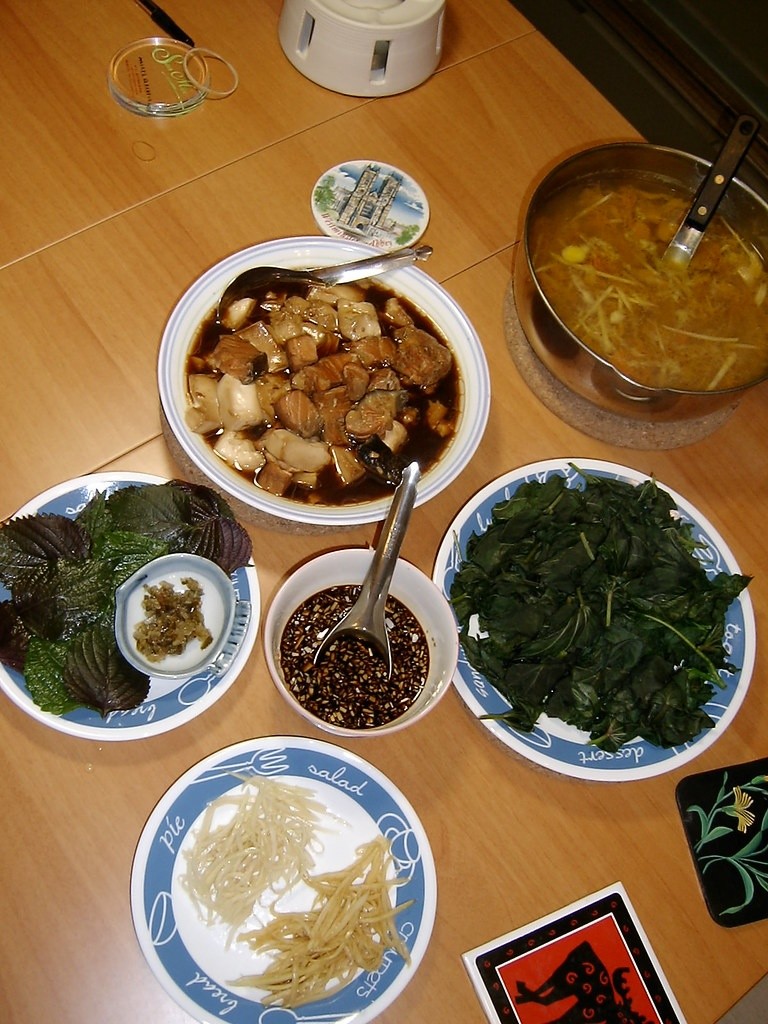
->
[313,461,420,681]
[216,245,433,331]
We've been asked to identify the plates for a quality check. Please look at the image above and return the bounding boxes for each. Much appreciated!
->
[432,457,756,781]
[130,735,437,1024]
[0,471,260,740]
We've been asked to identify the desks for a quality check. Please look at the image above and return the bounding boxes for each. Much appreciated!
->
[1,0,767,1024]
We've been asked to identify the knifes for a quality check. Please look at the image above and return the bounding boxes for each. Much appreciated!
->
[134,0,195,49]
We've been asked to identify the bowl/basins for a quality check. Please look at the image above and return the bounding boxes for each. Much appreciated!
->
[157,235,491,527]
[114,553,235,680]
[263,549,457,738]
[512,138,768,422]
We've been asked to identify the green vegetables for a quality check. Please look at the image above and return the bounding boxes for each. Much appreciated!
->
[0,479,255,720]
[446,460,754,752]
[530,191,760,391]
